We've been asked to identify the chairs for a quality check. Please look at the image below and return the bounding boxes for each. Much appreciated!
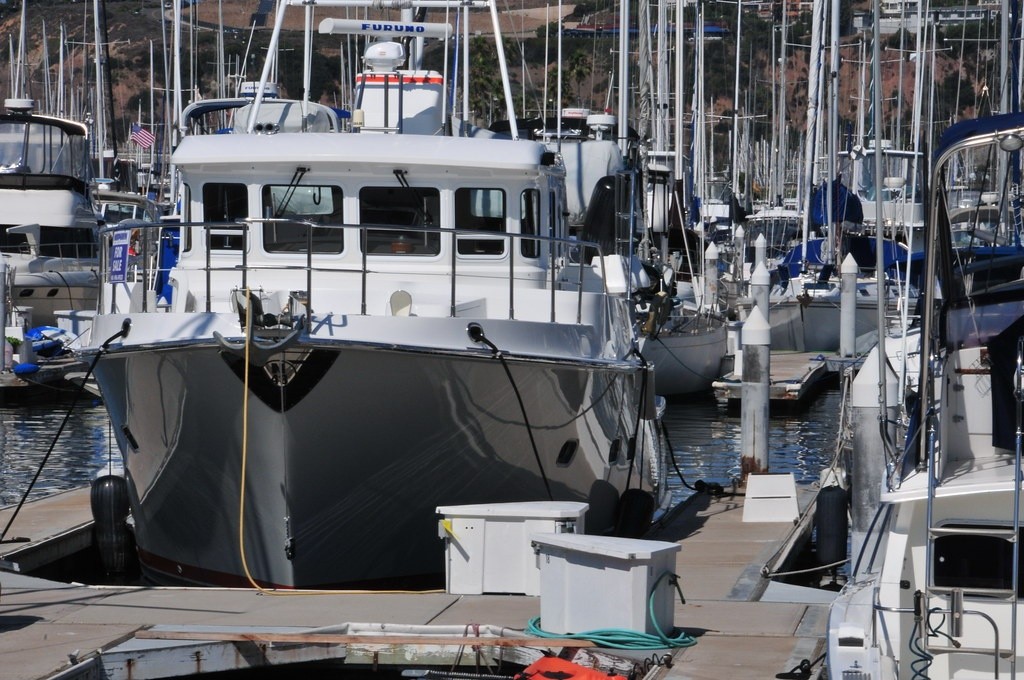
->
[269,207,539,258]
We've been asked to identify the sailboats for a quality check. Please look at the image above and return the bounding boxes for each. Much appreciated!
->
[0,0,1021,589]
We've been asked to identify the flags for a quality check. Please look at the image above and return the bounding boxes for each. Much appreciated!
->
[129,124,155,148]
